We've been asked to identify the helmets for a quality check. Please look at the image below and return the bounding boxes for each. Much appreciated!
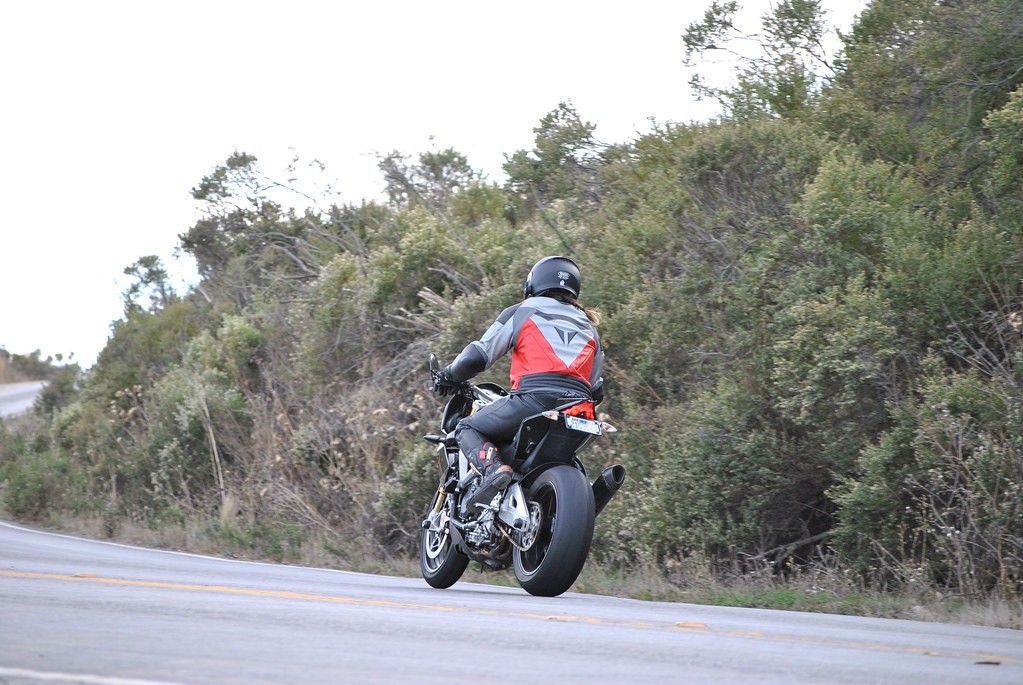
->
[522,255,582,299]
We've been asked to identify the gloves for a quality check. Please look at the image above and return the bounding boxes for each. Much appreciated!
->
[433,370,459,397]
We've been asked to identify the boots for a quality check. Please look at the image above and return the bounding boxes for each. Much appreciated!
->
[465,440,514,515]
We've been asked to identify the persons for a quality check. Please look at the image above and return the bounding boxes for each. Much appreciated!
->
[433,256,605,514]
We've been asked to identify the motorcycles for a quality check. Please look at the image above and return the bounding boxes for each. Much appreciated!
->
[419,353,626,596]
